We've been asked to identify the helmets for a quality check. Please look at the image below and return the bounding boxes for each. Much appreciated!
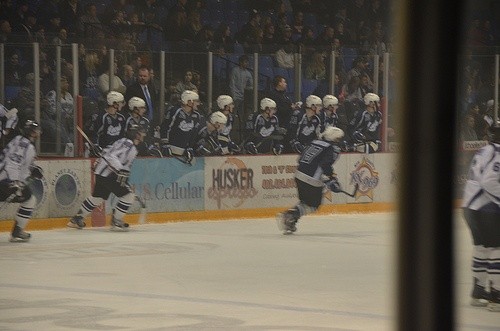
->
[181,90,199,104]
[363,93,380,105]
[107,90,124,105]
[323,95,338,107]
[127,97,145,110]
[209,111,227,125]
[130,123,147,141]
[323,127,344,142]
[260,97,277,109]
[19,119,38,134]
[217,95,233,110]
[305,94,323,108]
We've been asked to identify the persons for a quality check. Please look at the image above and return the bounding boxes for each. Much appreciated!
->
[89,90,127,158]
[0,120,44,243]
[126,97,154,157]
[0,0,393,140]
[275,127,344,235]
[198,95,241,156]
[67,117,149,232]
[348,93,382,153]
[243,97,284,155]
[290,94,338,154]
[463,119,500,312]
[464,20,500,140]
[161,90,201,155]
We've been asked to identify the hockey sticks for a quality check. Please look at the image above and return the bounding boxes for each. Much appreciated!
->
[153,143,194,166]
[78,127,149,223]
[332,182,361,198]
[195,98,386,155]
[0,165,44,210]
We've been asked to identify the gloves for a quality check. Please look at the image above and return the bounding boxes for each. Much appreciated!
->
[138,139,304,165]
[327,178,341,192]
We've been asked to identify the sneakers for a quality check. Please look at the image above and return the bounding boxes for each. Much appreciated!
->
[67,214,86,229]
[9,226,32,242]
[469,277,500,312]
[277,207,297,235]
[111,219,130,231]
